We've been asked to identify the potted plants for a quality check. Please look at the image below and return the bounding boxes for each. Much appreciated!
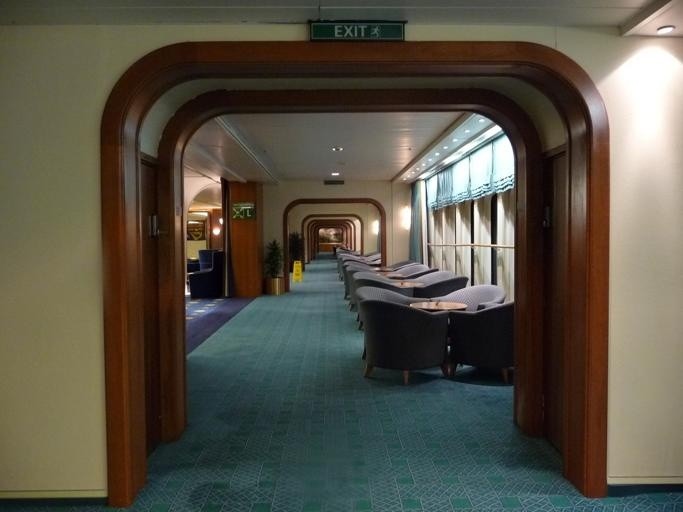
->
[264,239,286,294]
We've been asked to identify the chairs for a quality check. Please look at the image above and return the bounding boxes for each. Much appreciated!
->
[333,246,513,386]
[188,249,223,300]
